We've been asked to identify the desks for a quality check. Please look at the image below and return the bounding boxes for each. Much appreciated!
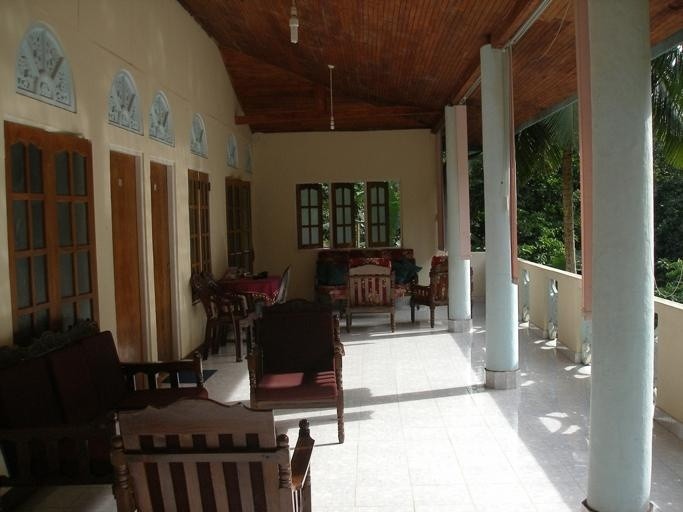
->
[328,288,406,319]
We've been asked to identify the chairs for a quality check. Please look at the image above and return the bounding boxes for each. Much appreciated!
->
[246,298,348,444]
[411,265,473,328]
[346,265,396,333]
[109,396,315,512]
[190,264,293,363]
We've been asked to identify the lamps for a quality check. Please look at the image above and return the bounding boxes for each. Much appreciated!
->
[327,65,335,130]
[288,0,300,44]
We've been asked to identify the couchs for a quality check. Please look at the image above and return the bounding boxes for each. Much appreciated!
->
[1,318,208,500]
[314,248,420,311]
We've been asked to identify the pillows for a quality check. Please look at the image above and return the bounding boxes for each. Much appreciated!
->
[316,256,423,285]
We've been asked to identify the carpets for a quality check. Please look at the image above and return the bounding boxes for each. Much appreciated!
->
[160,369,218,384]
